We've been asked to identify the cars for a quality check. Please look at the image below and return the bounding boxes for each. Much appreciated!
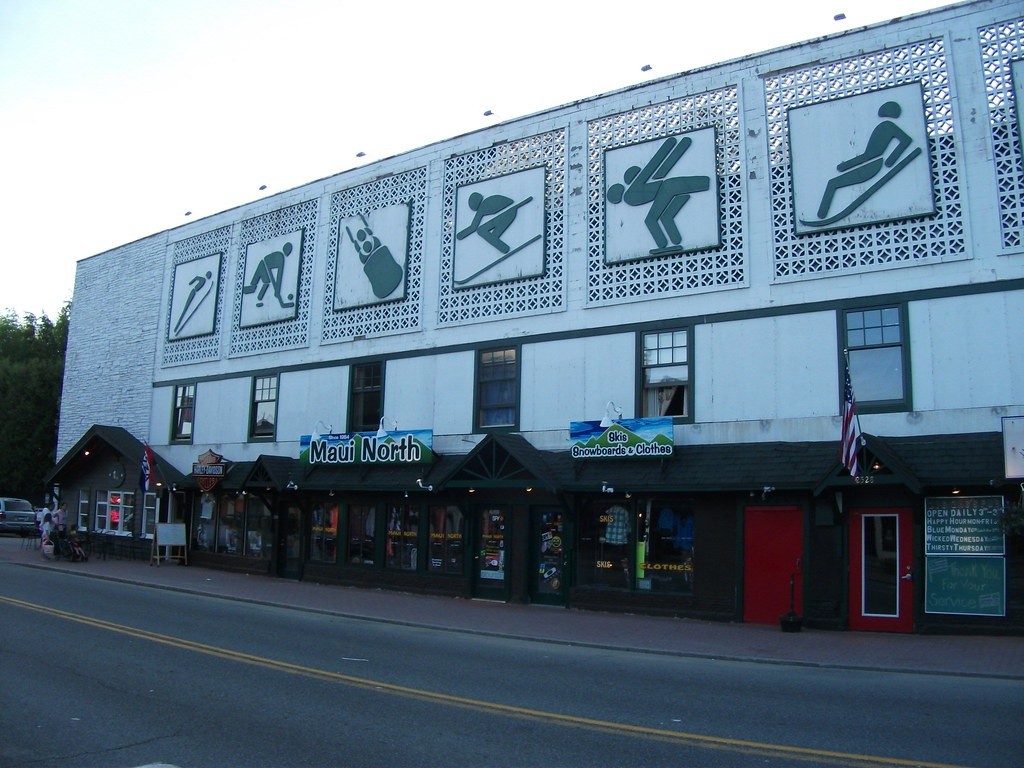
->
[0,497,37,538]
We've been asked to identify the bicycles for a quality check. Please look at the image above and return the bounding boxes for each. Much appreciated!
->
[55,522,94,561]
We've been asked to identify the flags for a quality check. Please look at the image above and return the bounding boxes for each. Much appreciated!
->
[839,364,858,479]
[141,444,154,491]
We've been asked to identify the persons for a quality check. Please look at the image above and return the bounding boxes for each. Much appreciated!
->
[54,503,68,549]
[36,510,42,526]
[38,502,53,550]
[42,513,52,545]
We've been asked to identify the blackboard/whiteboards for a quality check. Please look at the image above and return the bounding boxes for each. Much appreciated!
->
[153,523,186,546]
[925,555,1006,617]
[925,496,1005,555]
